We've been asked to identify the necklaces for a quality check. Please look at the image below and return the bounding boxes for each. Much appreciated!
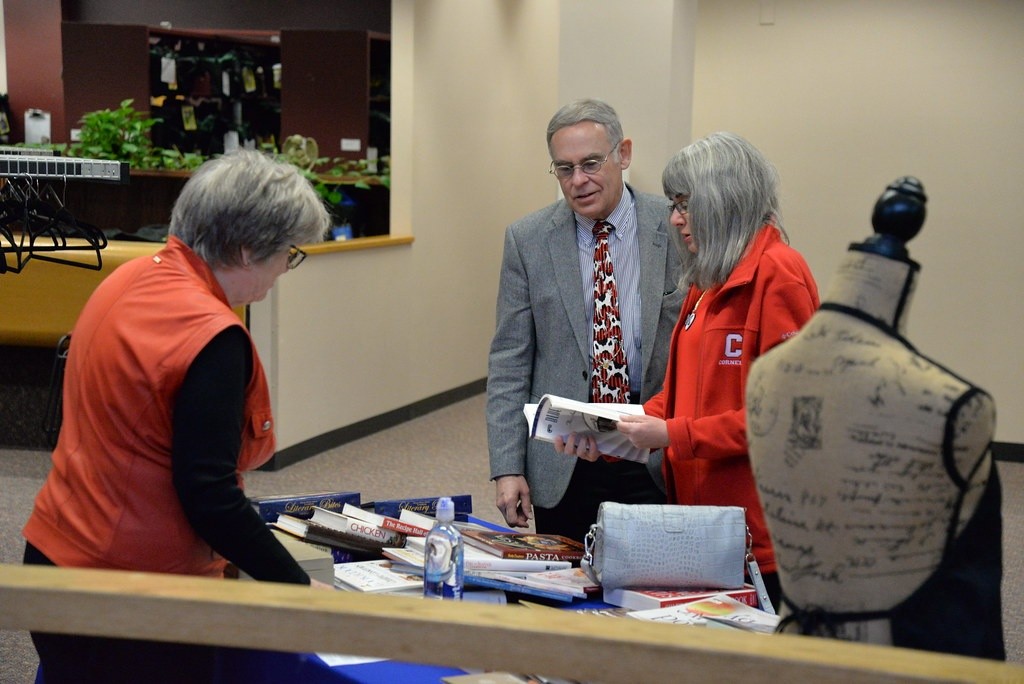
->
[685,290,705,329]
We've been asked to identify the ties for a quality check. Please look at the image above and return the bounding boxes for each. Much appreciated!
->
[592,222,631,431]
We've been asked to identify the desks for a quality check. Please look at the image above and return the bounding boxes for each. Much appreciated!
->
[33,514,623,684]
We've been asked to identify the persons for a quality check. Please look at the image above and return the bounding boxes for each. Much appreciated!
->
[554,132,821,614]
[486,99,689,568]
[23,150,333,684]
[744,176,1008,664]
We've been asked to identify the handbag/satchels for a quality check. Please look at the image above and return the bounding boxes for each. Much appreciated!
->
[580,501,776,614]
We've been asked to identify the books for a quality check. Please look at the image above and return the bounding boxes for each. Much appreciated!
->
[248,491,781,684]
[523,393,649,463]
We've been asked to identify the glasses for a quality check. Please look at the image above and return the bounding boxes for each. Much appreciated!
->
[667,199,689,215]
[549,142,618,179]
[287,244,308,269]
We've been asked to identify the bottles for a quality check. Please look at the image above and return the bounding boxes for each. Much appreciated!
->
[423,496,465,603]
